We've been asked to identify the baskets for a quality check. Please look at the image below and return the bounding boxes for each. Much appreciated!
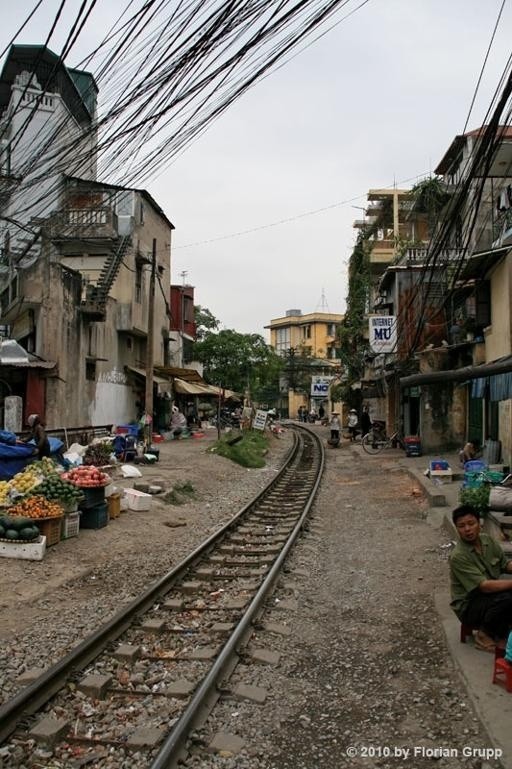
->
[63,514,80,538]
[35,519,62,546]
[404,437,421,457]
[79,503,108,528]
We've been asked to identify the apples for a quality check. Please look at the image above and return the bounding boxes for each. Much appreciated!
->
[61,465,109,485]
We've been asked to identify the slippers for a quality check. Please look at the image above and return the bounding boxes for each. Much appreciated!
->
[475,643,495,653]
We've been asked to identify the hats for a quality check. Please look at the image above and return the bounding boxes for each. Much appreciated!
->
[332,411,339,415]
[350,408,357,413]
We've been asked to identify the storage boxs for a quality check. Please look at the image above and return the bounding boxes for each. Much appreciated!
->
[1,534,47,562]
[119,487,153,511]
[428,459,453,484]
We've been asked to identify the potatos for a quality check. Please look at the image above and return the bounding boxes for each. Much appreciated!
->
[0,472,35,505]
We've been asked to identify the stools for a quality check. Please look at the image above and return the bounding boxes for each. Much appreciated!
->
[493,657,512,694]
[461,623,474,643]
[496,639,506,660]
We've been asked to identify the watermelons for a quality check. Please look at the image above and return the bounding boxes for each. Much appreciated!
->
[0,513,40,540]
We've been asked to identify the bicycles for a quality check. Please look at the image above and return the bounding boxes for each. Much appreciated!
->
[361,416,406,456]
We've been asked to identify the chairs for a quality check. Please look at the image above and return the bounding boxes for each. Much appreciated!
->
[122,434,139,464]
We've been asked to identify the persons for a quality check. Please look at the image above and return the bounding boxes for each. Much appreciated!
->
[448,504,512,651]
[329,410,341,439]
[459,439,480,463]
[346,408,358,443]
[302,405,308,422]
[21,415,50,460]
[360,407,371,439]
[319,406,324,419]
[298,406,303,421]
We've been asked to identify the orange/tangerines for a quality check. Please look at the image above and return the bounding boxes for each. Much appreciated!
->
[15,475,84,504]
